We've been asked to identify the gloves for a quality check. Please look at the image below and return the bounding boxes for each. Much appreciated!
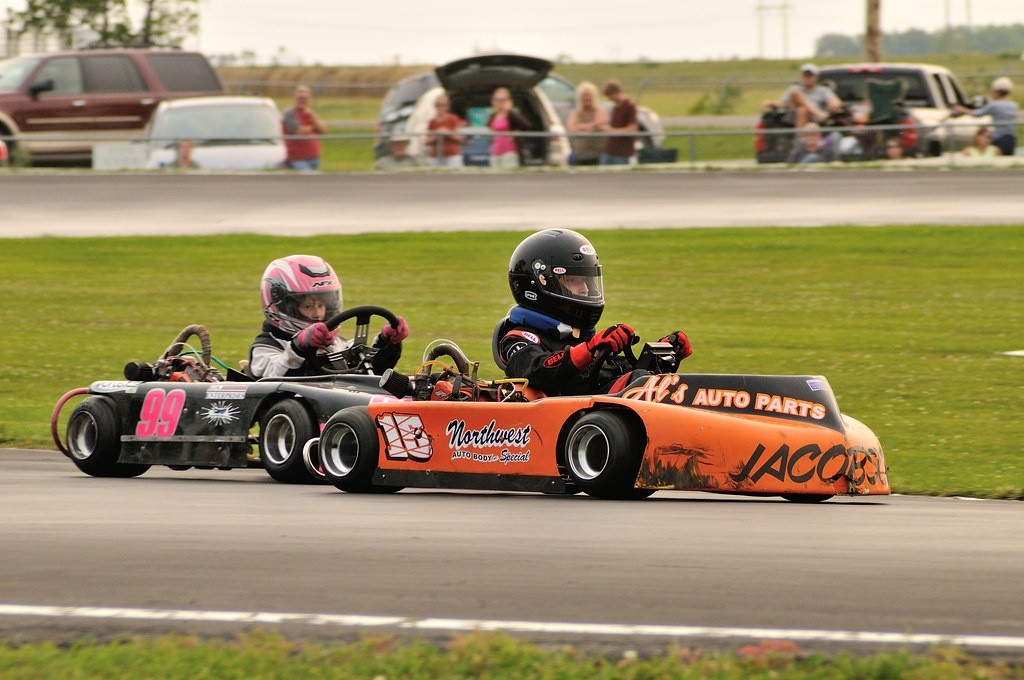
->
[382,316,409,345]
[298,323,337,350]
[569,324,640,369]
[658,331,693,361]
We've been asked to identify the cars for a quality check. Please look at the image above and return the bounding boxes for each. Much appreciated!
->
[392,55,572,168]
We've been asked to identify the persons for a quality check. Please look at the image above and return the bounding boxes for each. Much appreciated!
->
[490,87,532,166]
[567,81,637,165]
[249,254,408,380]
[425,96,468,167]
[500,229,693,405]
[282,85,323,169]
[953,76,1018,155]
[160,141,199,168]
[961,128,1002,157]
[762,63,915,167]
[373,135,417,169]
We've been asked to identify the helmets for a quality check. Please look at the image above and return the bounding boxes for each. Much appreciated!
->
[260,255,343,335]
[508,229,606,330]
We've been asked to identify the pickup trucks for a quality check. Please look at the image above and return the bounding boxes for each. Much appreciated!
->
[95,93,291,174]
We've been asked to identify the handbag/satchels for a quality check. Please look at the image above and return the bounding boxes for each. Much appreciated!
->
[634,105,665,151]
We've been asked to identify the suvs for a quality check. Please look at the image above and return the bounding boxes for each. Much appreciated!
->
[367,56,666,170]
[2,49,228,167]
[824,63,997,160]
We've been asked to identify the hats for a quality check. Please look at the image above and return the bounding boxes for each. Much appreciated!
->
[840,136,863,155]
[801,64,819,76]
